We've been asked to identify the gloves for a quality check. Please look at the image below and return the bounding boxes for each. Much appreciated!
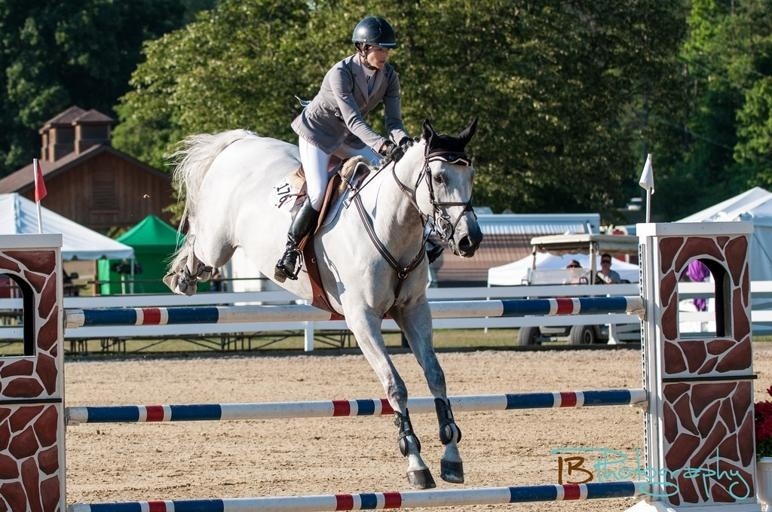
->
[385,143,404,162]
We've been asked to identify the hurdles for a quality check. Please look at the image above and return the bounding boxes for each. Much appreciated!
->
[0,221,761,510]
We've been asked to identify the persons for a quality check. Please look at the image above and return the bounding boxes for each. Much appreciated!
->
[562,259,584,284]
[595,253,621,284]
[281,16,420,277]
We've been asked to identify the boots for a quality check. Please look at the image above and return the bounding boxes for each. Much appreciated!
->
[274,196,319,283]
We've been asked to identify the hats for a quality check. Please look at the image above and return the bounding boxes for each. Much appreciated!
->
[566,260,582,268]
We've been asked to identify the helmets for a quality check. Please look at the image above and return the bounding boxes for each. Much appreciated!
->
[352,16,399,48]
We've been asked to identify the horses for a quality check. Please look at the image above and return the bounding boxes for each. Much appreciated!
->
[162,114,484,491]
